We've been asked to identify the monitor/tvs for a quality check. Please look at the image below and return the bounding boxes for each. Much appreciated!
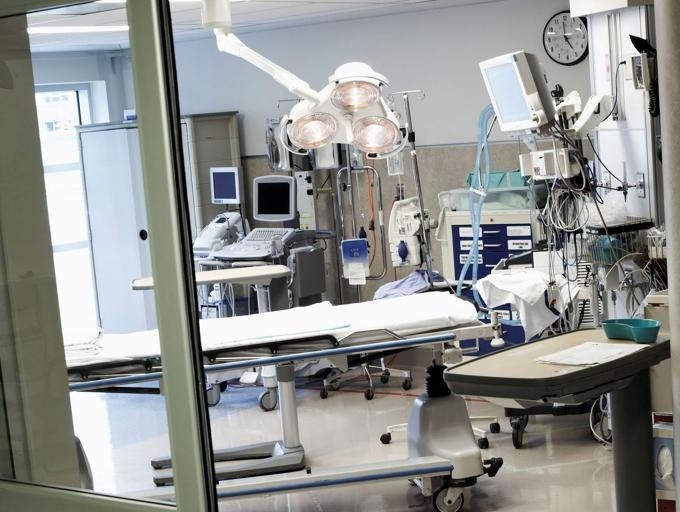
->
[251,174,295,222]
[477,50,550,132]
[210,166,240,203]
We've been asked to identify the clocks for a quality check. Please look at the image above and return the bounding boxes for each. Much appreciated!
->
[543,11,590,66]
